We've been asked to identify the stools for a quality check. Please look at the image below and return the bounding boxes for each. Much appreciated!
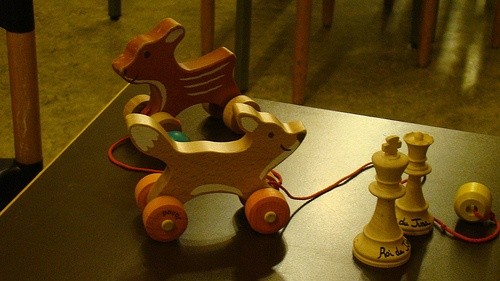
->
[101,0,443,108]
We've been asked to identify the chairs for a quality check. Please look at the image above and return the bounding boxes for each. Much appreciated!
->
[1,0,43,211]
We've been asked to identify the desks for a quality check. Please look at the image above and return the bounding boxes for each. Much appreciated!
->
[0,76,500,280]
